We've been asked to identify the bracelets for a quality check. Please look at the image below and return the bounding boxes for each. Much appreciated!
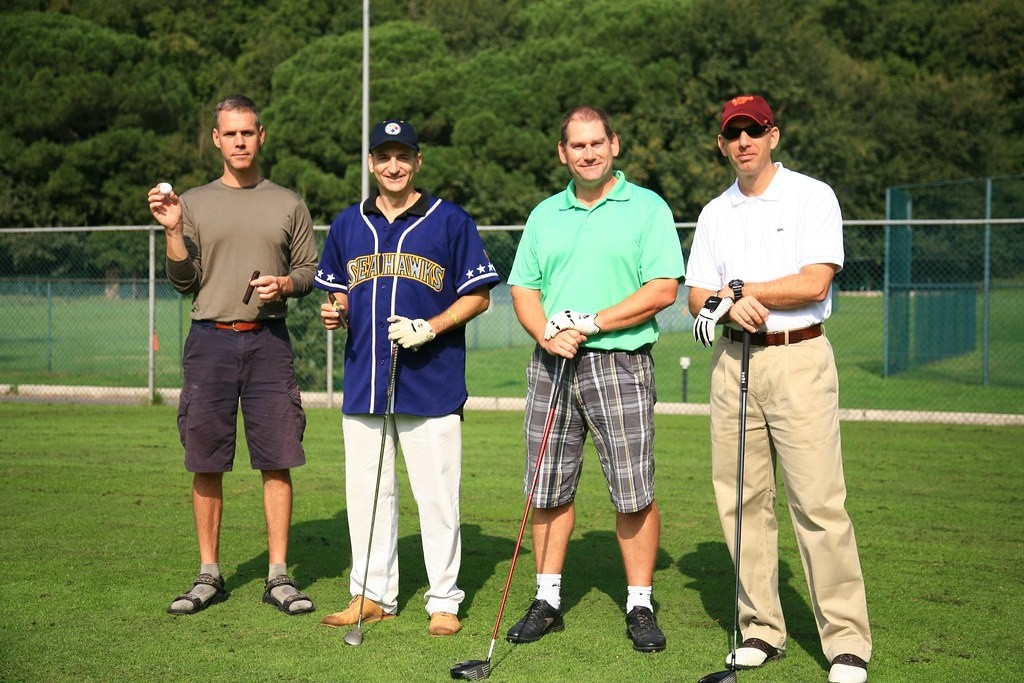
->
[445,310,460,328]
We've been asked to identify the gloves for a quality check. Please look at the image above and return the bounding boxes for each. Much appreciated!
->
[692,290,735,348]
[387,316,436,348]
[544,309,601,341]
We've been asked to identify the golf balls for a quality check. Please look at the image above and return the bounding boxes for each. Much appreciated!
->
[160,182,173,195]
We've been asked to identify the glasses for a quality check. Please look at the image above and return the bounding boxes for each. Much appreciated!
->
[721,124,767,140]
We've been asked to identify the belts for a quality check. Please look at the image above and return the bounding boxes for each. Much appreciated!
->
[723,325,822,346]
[216,322,262,331]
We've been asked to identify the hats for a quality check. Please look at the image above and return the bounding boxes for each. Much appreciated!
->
[721,95,774,132]
[369,120,419,150]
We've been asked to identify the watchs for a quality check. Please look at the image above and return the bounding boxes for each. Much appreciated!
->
[728,279,744,301]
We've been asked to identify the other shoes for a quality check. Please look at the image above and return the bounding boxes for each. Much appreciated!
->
[828,654,868,683]
[726,638,785,670]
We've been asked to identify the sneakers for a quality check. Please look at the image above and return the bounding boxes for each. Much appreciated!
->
[507,597,564,642]
[626,606,666,651]
[325,595,396,628]
[430,612,461,634]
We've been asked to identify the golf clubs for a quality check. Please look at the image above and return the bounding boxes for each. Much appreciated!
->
[696,328,753,683]
[450,354,569,682]
[343,339,400,646]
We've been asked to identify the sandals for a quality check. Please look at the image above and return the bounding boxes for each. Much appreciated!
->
[167,574,227,614]
[262,575,315,614]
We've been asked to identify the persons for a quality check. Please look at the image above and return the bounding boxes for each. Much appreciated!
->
[505,107,687,652]
[685,95,876,683]
[147,95,319,615]
[313,118,500,636]
